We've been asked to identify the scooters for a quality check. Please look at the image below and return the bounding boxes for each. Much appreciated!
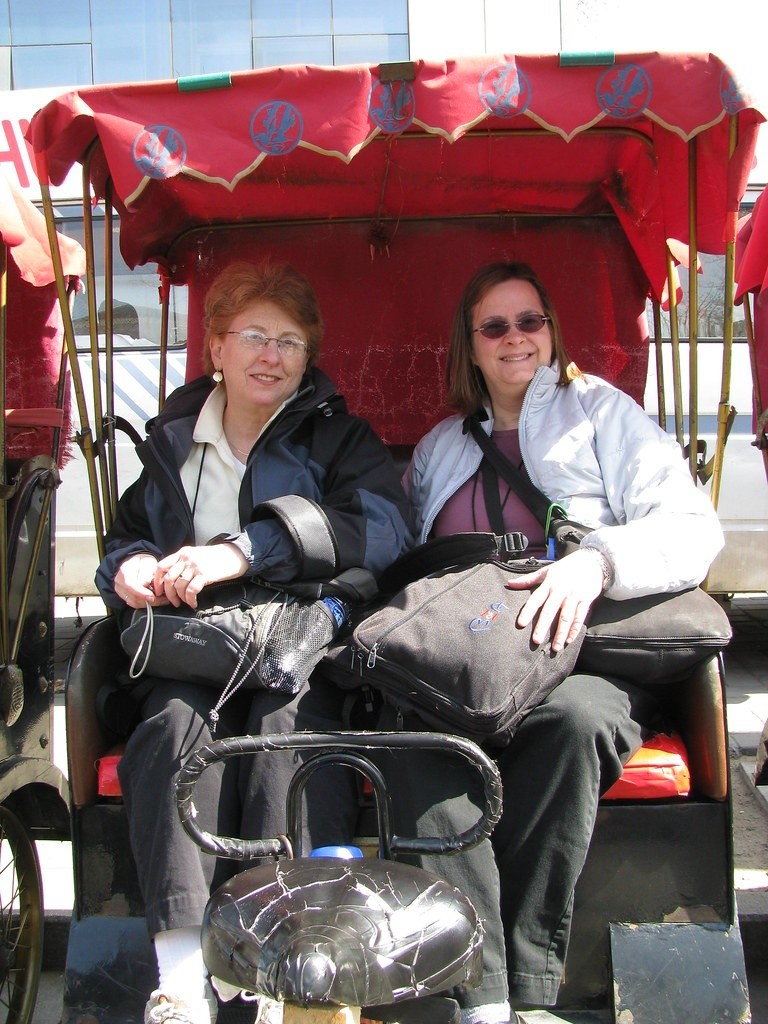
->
[1,160,149,1023]
[24,45,768,1021]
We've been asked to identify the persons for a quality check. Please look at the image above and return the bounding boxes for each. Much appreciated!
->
[92,261,407,1024]
[387,260,726,1024]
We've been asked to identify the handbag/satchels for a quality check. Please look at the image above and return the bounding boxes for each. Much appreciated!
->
[555,521,733,685]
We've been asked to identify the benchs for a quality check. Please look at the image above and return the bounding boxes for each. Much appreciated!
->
[66,441,732,809]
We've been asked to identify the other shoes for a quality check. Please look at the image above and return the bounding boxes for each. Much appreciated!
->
[144,989,218,1024]
[242,989,284,1024]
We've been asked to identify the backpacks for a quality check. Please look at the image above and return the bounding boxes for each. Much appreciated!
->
[119,493,378,697]
[313,531,586,751]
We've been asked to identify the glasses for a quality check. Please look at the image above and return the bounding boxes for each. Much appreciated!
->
[472,313,550,339]
[218,328,314,356]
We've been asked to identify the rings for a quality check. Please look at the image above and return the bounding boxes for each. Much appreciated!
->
[124,594,129,601]
[178,574,191,582]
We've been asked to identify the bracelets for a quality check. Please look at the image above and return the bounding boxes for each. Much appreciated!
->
[582,546,611,590]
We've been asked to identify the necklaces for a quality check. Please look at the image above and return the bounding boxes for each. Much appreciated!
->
[229,440,248,456]
